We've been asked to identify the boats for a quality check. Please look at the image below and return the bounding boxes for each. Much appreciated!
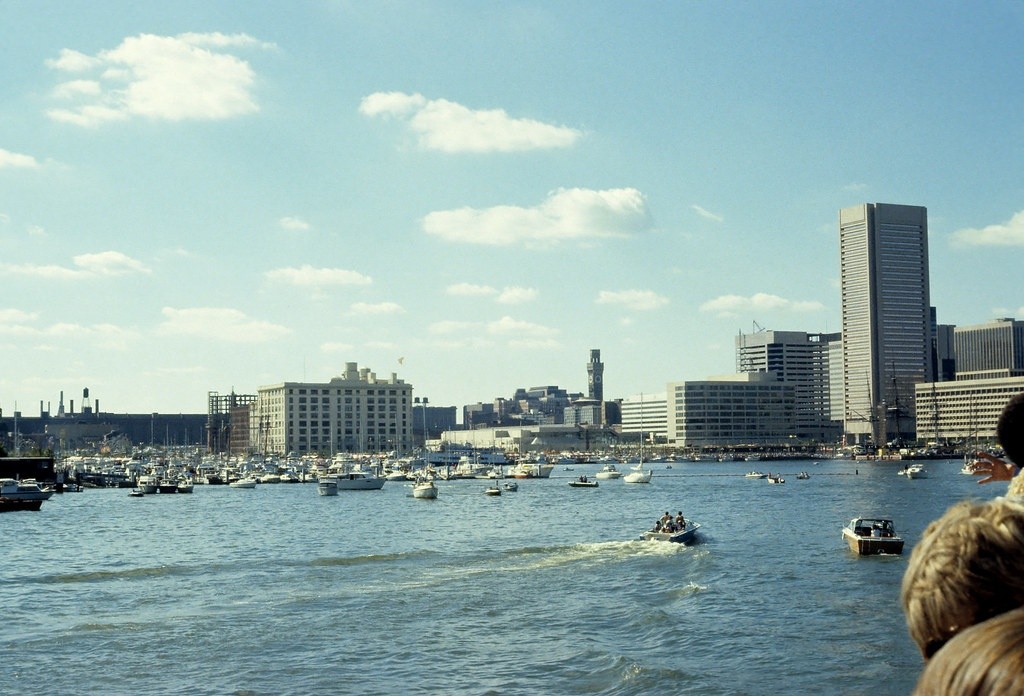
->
[623,472,652,483]
[136,475,194,494]
[55,456,136,488]
[906,464,928,478]
[568,475,599,487]
[897,470,907,476]
[767,473,785,484]
[961,459,982,475]
[229,476,256,488]
[798,475,810,480]
[0,478,57,500]
[128,489,144,497]
[0,497,43,512]
[563,467,574,471]
[900,382,1007,461]
[842,517,905,557]
[136,455,194,474]
[595,465,622,479]
[195,451,555,499]
[744,470,768,479]
[54,484,84,492]
[638,517,701,544]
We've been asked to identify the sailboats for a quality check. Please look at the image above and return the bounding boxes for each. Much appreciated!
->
[629,393,643,470]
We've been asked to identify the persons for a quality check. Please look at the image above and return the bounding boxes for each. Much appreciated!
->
[655,511,685,533]
[871,523,892,537]
[580,475,587,482]
[898,391,1024,696]
[801,471,807,476]
